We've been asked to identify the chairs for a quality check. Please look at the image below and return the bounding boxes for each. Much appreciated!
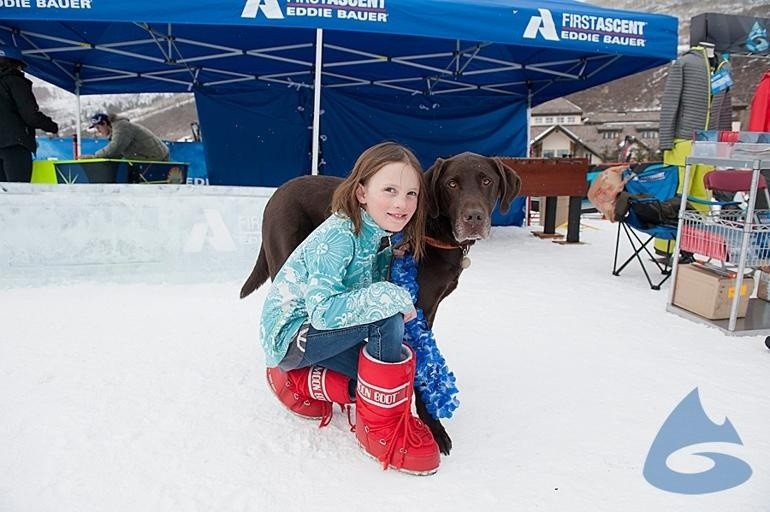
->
[613,162,696,290]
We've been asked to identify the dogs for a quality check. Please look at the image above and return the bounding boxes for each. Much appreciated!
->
[239,148,522,456]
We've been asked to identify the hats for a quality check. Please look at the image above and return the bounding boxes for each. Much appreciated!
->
[0,45,29,70]
[89,113,109,128]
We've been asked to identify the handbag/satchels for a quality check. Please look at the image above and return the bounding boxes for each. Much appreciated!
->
[587,161,641,222]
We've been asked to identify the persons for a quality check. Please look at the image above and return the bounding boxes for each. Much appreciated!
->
[75,112,169,162]
[0,42,60,184]
[651,42,734,262]
[258,139,442,475]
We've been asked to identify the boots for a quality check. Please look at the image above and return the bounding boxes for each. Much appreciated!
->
[266,362,357,422]
[353,339,441,476]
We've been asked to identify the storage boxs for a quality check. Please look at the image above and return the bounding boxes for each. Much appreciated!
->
[673,263,754,321]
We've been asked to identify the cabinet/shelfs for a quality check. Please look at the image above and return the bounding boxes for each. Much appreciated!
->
[666,154,769,338]
